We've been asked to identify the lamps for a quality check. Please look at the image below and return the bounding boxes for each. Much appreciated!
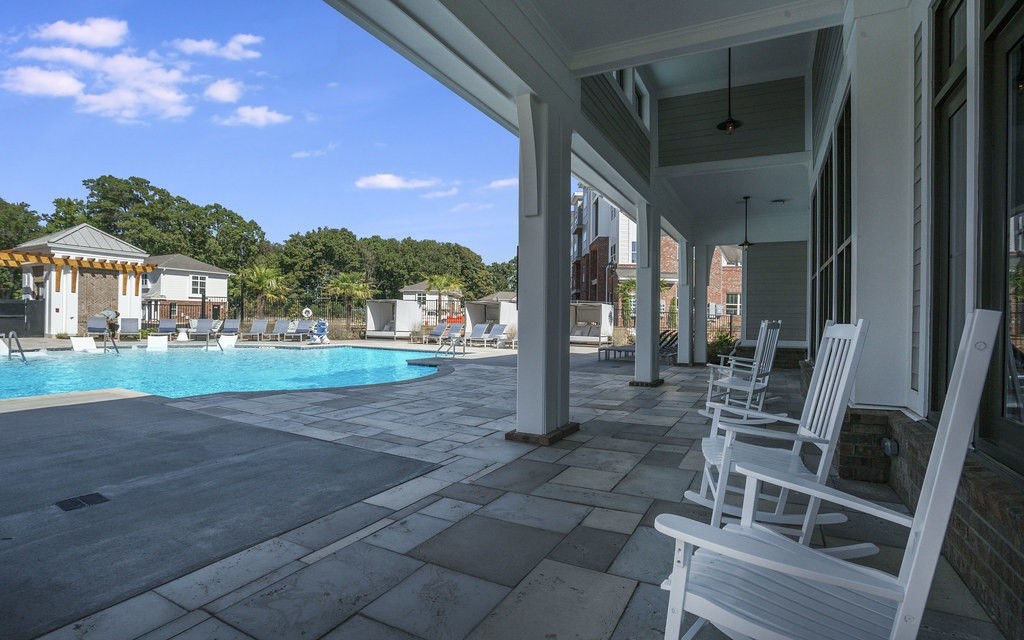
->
[717,47,742,134]
[738,196,754,251]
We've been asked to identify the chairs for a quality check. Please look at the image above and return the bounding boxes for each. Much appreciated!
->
[426,323,518,348]
[189,318,328,343]
[117,317,142,341]
[148,318,177,342]
[599,310,1003,640]
[86,316,109,341]
